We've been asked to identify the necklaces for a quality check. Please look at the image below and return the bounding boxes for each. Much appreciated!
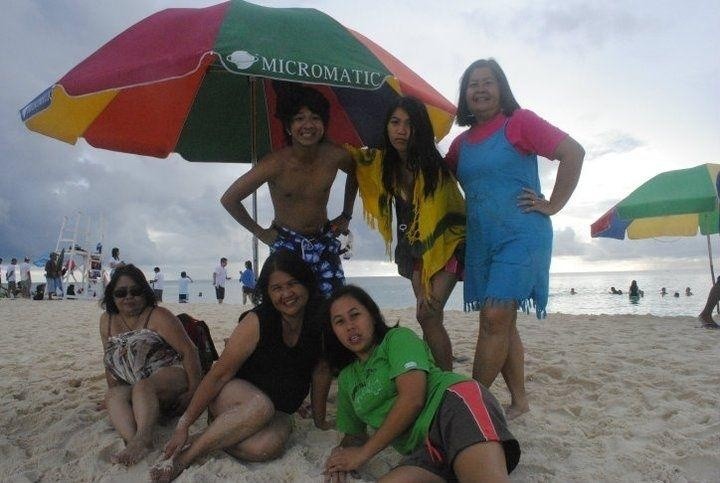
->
[120,307,145,331]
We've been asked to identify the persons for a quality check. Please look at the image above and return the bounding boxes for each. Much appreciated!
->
[568,274,695,304]
[697,276,719,327]
[1,247,260,304]
[442,58,588,412]
[340,96,468,373]
[221,86,358,293]
[100,259,201,467]
[153,245,335,483]
[326,284,521,483]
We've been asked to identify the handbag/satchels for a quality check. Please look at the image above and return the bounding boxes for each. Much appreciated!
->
[177,313,219,372]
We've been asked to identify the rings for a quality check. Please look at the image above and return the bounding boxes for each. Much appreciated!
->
[532,200,536,207]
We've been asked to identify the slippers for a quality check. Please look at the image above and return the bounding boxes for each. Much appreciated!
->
[694,321,720,330]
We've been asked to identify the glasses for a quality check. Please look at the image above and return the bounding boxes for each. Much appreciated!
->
[113,288,142,297]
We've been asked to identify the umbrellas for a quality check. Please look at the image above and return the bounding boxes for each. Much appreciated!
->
[590,161,719,325]
[20,0,460,277]
